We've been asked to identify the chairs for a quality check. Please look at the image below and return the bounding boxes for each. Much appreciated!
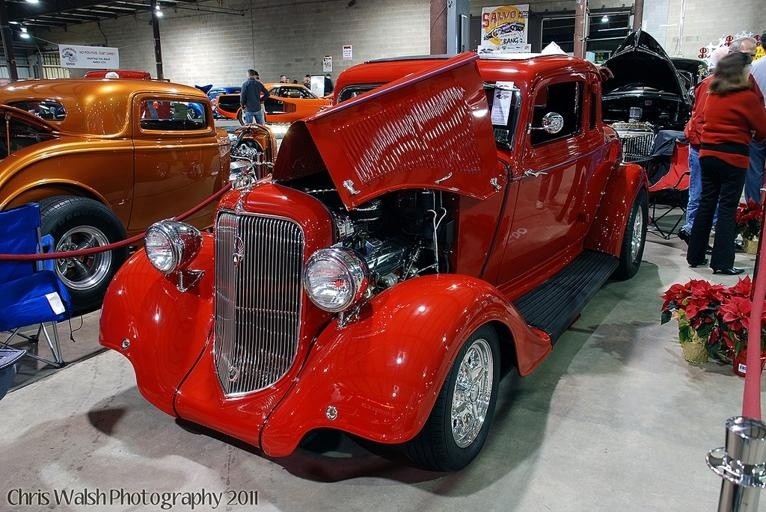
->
[630,137,690,240]
[0,201,73,369]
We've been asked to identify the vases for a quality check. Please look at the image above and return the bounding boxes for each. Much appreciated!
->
[678,330,708,365]
[733,344,766,377]
[743,236,759,255]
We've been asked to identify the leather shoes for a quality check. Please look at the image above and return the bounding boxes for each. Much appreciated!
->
[676,227,748,273]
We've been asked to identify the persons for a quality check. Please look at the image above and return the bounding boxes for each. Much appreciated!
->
[744,29,766,204]
[536,165,564,209]
[677,37,765,257]
[253,71,264,124]
[556,156,591,224]
[279,74,333,97]
[686,51,766,275]
[240,69,270,124]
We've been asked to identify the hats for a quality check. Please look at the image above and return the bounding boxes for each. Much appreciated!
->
[248,70,259,76]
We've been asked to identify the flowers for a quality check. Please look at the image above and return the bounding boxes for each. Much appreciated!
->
[734,197,763,240]
[720,274,766,354]
[657,277,720,361]
